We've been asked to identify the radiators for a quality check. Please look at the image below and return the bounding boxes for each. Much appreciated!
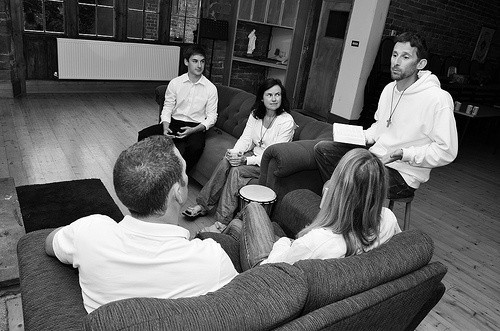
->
[55,37,181,81]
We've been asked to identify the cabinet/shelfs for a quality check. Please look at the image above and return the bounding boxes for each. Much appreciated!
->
[222,0,312,88]
[445,81,500,106]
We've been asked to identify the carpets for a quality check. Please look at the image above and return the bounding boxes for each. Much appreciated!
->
[15,179,124,232]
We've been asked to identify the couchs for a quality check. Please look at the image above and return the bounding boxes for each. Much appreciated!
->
[16,189,447,331]
[156,81,334,215]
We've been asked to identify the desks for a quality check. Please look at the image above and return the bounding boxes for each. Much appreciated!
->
[453,101,500,154]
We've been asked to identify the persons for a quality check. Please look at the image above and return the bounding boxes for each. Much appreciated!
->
[45,135,239,314]
[221,148,402,272]
[313,32,458,199]
[182,78,300,235]
[138,45,218,185]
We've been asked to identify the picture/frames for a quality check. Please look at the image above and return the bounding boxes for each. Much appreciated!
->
[470,26,496,63]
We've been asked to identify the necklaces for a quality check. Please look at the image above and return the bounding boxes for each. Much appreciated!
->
[258,115,276,147]
[386,78,418,128]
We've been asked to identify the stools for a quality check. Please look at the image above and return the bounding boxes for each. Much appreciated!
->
[389,191,415,231]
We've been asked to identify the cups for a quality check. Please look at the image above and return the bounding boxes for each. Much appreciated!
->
[472,106,480,115]
[454,101,461,111]
[466,105,473,114]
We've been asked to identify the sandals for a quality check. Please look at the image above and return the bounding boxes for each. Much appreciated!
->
[182,204,206,217]
[196,221,228,236]
